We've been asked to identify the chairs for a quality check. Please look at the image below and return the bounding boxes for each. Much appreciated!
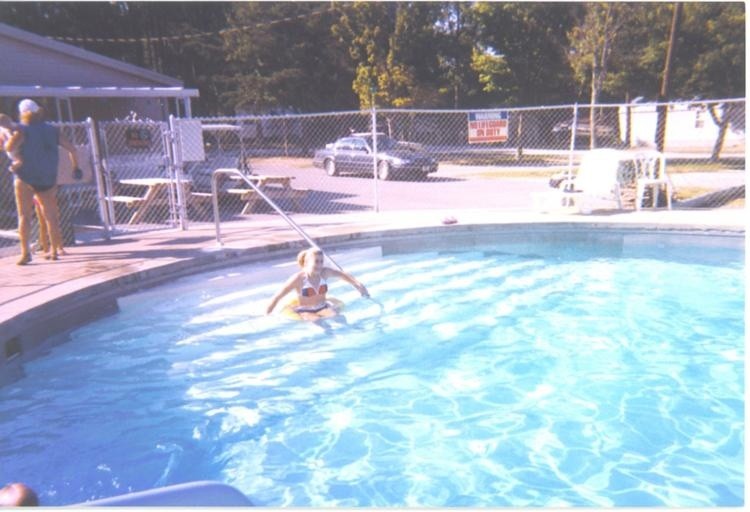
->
[557,149,624,213]
[633,148,673,210]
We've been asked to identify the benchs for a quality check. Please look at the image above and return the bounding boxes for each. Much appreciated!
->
[105,176,309,227]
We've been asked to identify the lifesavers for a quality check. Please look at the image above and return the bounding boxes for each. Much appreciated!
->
[283,295,345,321]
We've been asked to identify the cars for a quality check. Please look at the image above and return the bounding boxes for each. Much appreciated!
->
[551,119,612,143]
[311,132,439,180]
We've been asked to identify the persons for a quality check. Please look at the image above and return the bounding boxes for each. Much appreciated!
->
[32,191,69,256]
[4,98,82,265]
[263,246,369,322]
[0,113,25,173]
[0,481,38,506]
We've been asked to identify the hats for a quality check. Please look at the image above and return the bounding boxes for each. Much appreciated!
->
[18,99,39,113]
